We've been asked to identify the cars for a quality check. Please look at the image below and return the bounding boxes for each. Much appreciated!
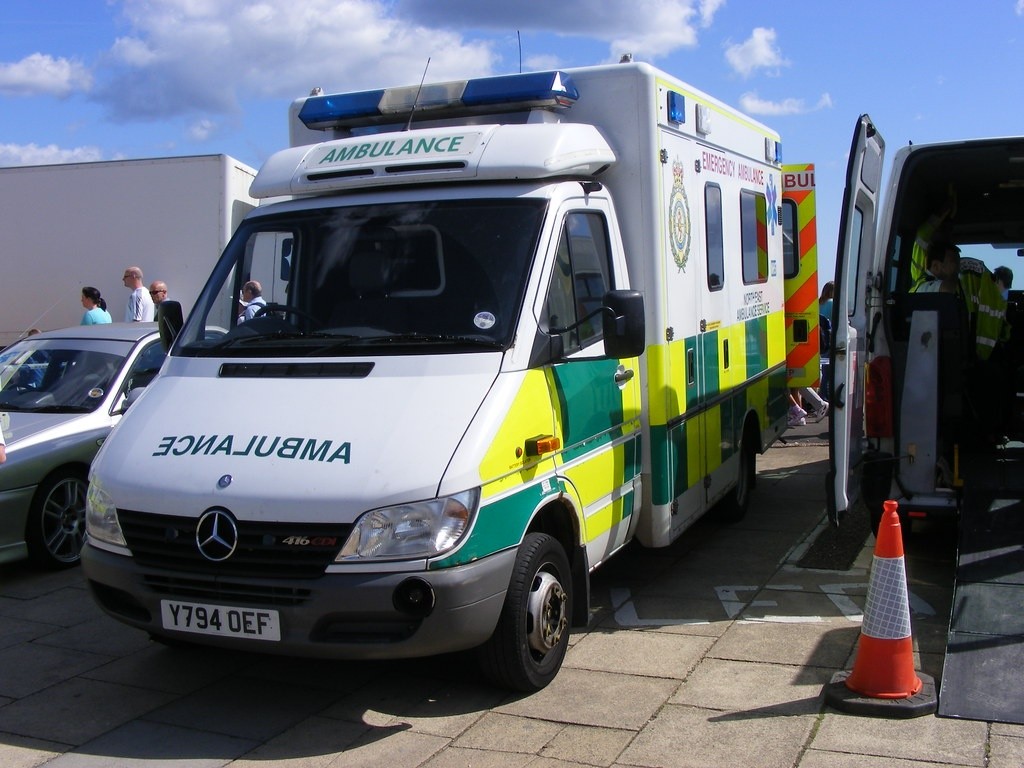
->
[0,321,231,573]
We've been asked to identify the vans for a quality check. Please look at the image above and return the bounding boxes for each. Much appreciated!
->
[825,114,1024,541]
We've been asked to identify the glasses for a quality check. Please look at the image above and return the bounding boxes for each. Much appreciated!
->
[150,291,164,295]
[124,276,129,279]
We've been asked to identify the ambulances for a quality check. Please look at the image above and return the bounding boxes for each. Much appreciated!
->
[85,53,818,693]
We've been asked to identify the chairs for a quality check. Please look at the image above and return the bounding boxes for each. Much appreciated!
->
[888,289,971,362]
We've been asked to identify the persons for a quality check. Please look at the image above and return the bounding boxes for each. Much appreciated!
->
[80,286,112,326]
[149,280,169,321]
[787,215,1024,443]
[123,267,153,322]
[20,329,53,391]
[236,281,267,326]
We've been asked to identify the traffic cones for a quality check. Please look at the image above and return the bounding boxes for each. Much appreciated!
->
[823,499,940,719]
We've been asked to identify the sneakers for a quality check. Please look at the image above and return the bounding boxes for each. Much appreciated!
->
[794,418,806,425]
[788,409,808,425]
[816,401,829,423]
